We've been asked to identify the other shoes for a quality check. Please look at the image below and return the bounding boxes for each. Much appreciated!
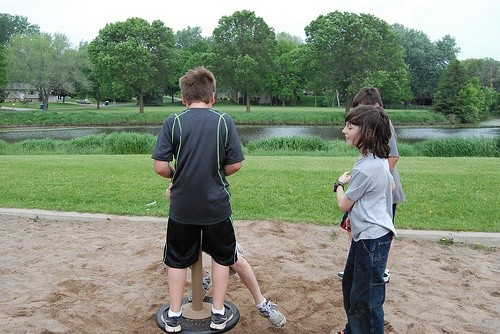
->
[383,268,390,283]
[338,272,344,280]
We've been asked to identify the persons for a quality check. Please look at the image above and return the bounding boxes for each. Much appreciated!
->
[151,67,286,332]
[333,88,404,334]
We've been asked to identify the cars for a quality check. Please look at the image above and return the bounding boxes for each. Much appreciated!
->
[76,98,91,105]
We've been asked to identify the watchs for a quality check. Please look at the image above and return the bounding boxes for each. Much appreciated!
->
[334,181,344,192]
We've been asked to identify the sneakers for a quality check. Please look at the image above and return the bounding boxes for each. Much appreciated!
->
[161,307,182,332]
[188,271,211,302]
[256,298,286,328]
[210,304,234,330]
